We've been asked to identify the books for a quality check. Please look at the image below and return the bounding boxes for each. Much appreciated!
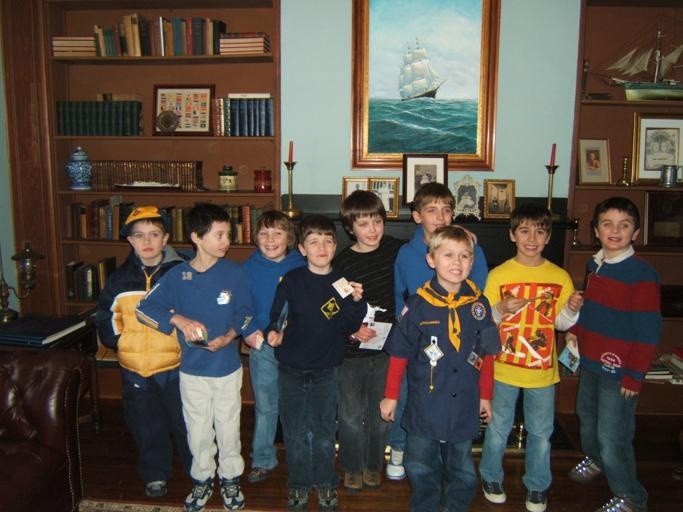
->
[51,13,272,57]
[61,202,252,302]
[359,322,392,350]
[647,346,682,387]
[0,317,86,346]
[56,93,144,135]
[211,93,275,137]
[91,159,203,191]
[558,339,581,373]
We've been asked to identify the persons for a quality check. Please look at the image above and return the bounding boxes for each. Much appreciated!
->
[135,201,254,512]
[96,207,193,498]
[331,190,477,492]
[241,209,304,483]
[454,183,481,211]
[385,181,488,481]
[380,226,501,511]
[264,212,366,511]
[587,152,599,169]
[492,190,506,212]
[350,185,362,193]
[565,195,662,512]
[479,203,583,511]
[380,185,392,210]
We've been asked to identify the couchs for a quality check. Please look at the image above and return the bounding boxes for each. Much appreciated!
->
[0,347,92,512]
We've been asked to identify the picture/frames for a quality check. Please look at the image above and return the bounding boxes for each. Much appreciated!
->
[403,154,448,208]
[450,174,515,223]
[352,0,501,172]
[631,110,683,185]
[151,85,215,135]
[579,138,612,186]
[342,176,400,219]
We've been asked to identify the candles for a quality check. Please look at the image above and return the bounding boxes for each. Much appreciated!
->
[289,141,293,162]
[550,144,556,166]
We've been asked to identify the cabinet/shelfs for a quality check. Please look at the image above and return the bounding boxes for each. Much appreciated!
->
[1,0,281,405]
[555,1,682,415]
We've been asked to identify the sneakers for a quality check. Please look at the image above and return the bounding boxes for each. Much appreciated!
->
[247,467,273,483]
[362,472,381,488]
[145,480,167,497]
[344,472,363,492]
[569,456,603,483]
[671,464,683,481]
[219,477,245,512]
[385,449,406,480]
[318,489,339,512]
[288,487,310,512]
[596,488,648,512]
[184,476,214,512]
[526,491,547,512]
[482,482,507,504]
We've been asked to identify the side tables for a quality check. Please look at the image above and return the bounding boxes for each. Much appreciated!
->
[0,314,100,427]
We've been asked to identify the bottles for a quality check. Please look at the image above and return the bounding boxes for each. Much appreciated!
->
[255,170,272,192]
[217,166,237,192]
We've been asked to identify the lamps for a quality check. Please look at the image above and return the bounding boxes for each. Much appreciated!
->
[0,242,43,323]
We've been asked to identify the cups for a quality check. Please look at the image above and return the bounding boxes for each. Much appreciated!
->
[660,164,683,188]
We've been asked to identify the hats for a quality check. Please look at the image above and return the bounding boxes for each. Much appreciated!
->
[119,206,168,237]
